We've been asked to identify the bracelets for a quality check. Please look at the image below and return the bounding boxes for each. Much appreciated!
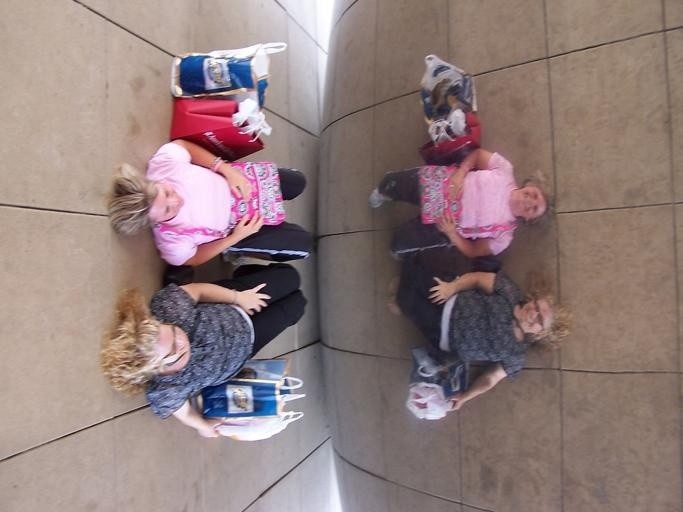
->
[211,158,223,172]
[232,289,237,302]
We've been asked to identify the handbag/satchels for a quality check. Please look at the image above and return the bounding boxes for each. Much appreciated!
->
[406,348,467,419]
[228,161,286,226]
[171,40,288,163]
[417,54,480,164]
[417,165,462,225]
[201,358,305,441]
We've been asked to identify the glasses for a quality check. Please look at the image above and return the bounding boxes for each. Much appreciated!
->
[162,323,177,360]
[534,301,544,330]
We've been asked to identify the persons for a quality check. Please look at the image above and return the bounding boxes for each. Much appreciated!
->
[101,262,308,437]
[368,148,554,258]
[107,140,311,266]
[388,260,575,411]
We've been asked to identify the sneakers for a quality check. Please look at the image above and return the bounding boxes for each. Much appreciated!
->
[387,276,403,316]
[368,187,385,209]
